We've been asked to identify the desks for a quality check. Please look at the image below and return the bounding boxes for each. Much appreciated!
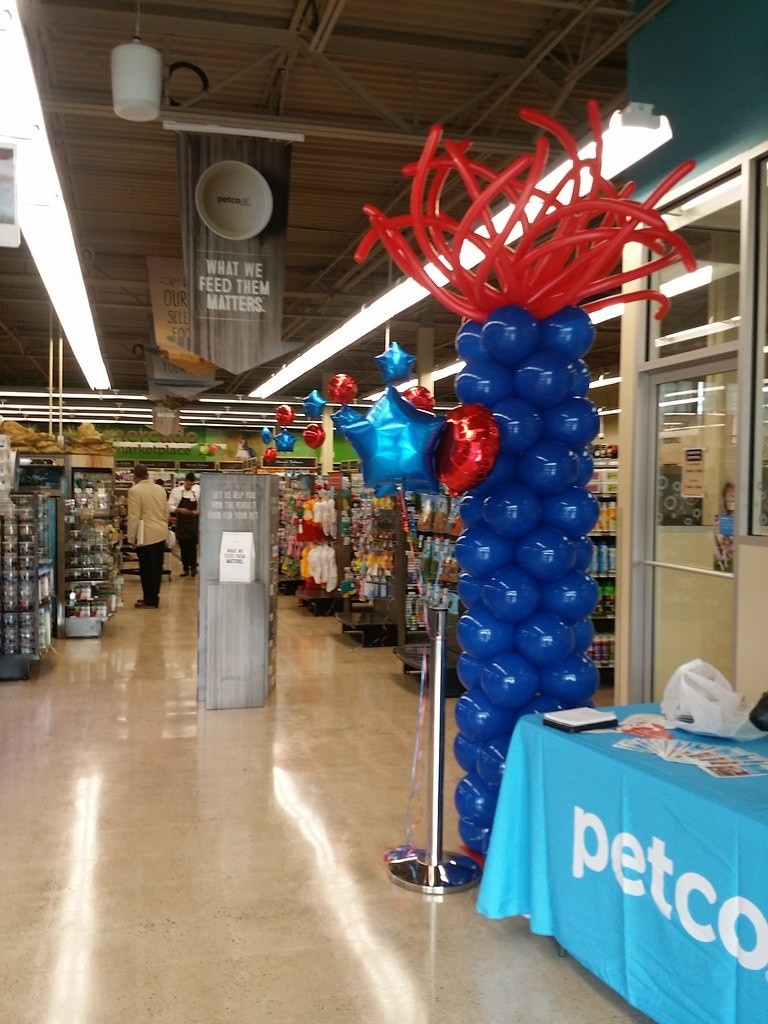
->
[477,704,768,1024]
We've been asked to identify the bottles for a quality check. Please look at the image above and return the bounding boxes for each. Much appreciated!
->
[583,500,617,664]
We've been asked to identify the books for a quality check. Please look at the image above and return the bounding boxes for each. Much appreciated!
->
[129,520,143,548]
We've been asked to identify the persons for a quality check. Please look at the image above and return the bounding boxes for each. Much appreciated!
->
[126,464,170,610]
[167,472,202,578]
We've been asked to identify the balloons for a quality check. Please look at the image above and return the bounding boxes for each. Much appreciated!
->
[441,403,499,500]
[375,340,419,385]
[332,405,365,434]
[261,427,277,464]
[340,387,446,498]
[447,305,602,851]
[403,387,438,411]
[299,390,327,449]
[274,405,298,453]
[354,99,698,322]
[326,373,358,404]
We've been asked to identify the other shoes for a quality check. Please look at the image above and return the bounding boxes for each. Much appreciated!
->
[180,571,189,577]
[135,602,157,609]
[136,600,145,604]
[190,570,198,577]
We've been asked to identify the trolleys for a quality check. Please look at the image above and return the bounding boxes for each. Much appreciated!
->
[113,515,177,582]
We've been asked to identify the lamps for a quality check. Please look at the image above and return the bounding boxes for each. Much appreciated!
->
[109,0,162,121]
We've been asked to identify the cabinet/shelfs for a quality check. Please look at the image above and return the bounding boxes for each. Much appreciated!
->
[279,452,618,694]
[0,445,200,680]
